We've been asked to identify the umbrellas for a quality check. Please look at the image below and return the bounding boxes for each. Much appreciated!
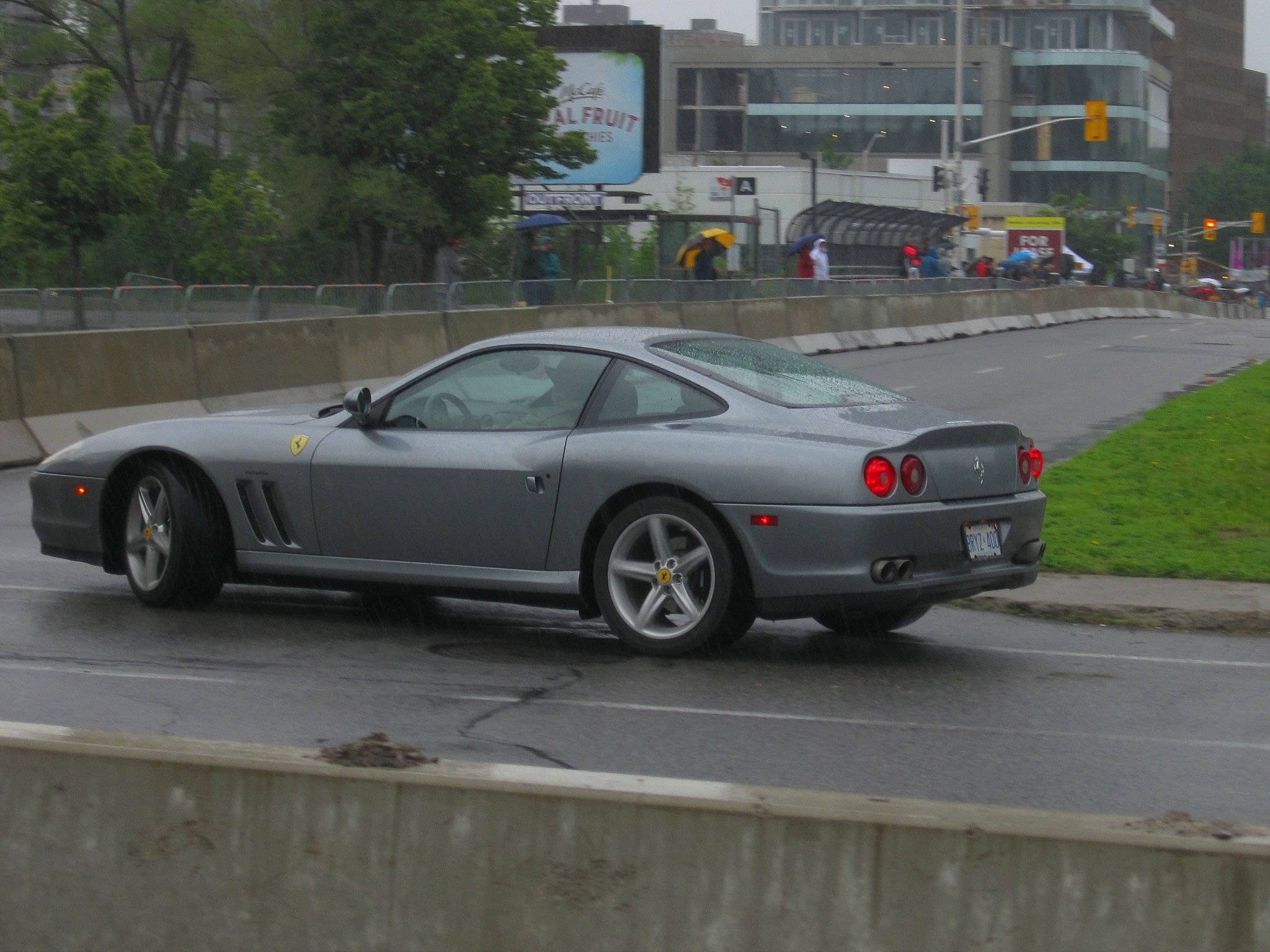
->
[1183,287,1213,296]
[515,212,572,231]
[786,234,825,258]
[966,256,995,275]
[998,259,1030,270]
[1199,278,1223,287]
[1008,251,1034,261]
[675,228,738,270]
[1207,295,1221,301]
[937,240,961,250]
[1039,253,1075,273]
[1234,288,1251,293]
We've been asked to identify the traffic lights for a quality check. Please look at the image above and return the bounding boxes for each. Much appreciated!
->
[932,164,944,193]
[978,168,991,196]
[1126,205,1138,229]
[1202,219,1217,241]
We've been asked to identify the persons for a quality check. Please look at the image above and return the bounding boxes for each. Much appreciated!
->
[798,241,818,295]
[694,237,721,281]
[518,225,562,305]
[1152,268,1265,310]
[808,238,831,296]
[423,225,466,306]
[898,235,1071,292]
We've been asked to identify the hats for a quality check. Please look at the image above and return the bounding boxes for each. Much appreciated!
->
[539,236,553,245]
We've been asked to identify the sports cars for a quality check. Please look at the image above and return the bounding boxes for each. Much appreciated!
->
[26,322,1046,660]
